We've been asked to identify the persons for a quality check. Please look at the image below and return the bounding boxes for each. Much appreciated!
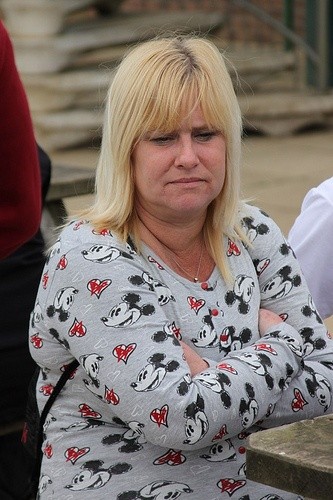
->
[28,33,333,500]
[0,21,50,500]
[286,176,333,319]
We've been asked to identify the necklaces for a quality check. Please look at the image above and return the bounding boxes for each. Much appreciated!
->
[170,238,205,283]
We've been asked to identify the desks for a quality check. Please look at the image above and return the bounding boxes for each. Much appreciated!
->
[245,412,333,500]
[43,165,97,228]
